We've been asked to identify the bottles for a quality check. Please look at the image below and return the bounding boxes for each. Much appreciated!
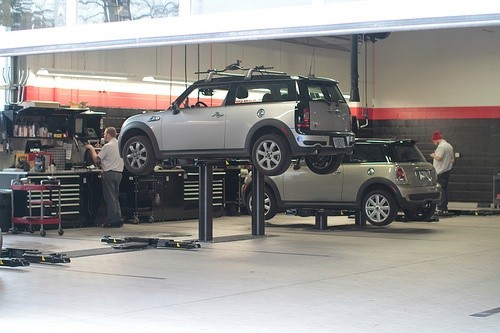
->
[51,162,54,172]
[14,118,66,138]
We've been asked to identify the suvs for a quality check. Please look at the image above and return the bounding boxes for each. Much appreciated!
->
[115,63,356,177]
[239,135,445,229]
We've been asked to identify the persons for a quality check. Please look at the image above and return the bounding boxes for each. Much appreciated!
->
[86,127,124,228]
[429,131,454,214]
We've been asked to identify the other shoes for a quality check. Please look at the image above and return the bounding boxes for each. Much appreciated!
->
[433,209,448,214]
[102,222,123,228]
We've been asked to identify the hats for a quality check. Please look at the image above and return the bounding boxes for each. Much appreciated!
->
[432,131,443,140]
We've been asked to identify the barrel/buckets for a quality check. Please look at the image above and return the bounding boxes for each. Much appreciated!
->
[34,154,45,172]
